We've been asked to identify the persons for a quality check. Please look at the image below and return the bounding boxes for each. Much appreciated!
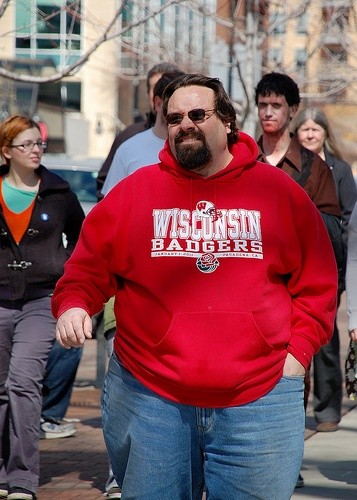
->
[247,73,340,488]
[50,73,339,500]
[294,107,357,432]
[0,114,86,500]
[95,63,194,500]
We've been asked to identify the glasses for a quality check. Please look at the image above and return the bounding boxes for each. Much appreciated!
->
[166,109,216,125]
[9,142,46,150]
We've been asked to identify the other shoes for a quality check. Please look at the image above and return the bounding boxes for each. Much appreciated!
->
[0,482,10,499]
[108,487,123,500]
[7,487,34,500]
[316,421,340,432]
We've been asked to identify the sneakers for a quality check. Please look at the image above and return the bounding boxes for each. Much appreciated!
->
[38,418,76,440]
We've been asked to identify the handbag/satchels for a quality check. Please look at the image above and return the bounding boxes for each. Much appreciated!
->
[344,340,357,401]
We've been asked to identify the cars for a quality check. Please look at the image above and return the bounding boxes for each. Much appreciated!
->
[39,153,106,218]
